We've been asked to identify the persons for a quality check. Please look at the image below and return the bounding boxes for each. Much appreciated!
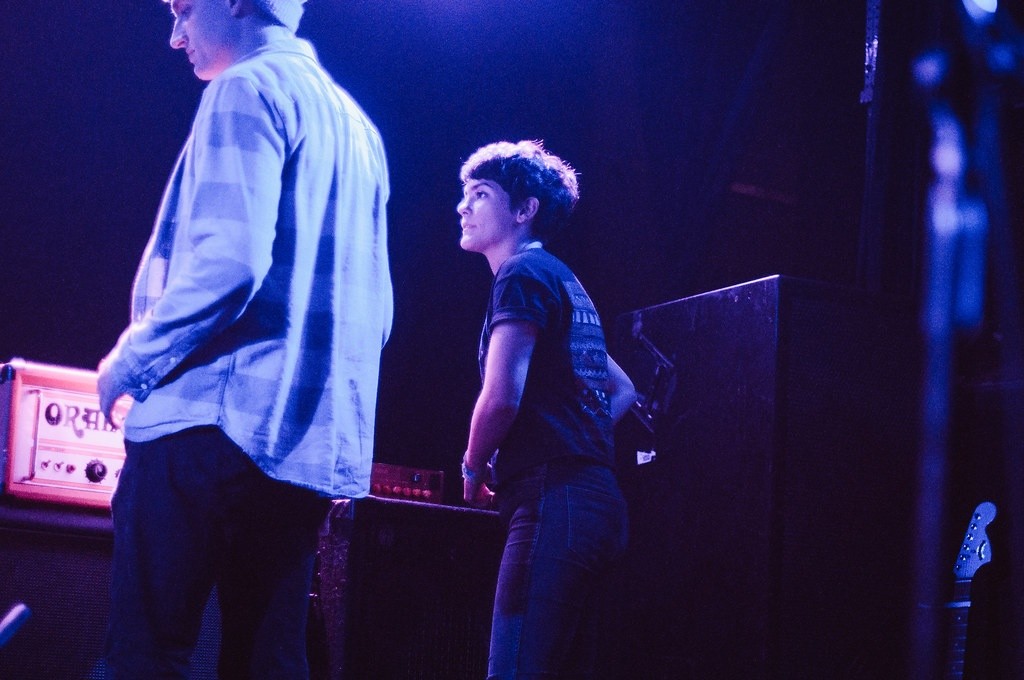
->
[458,142,635,676]
[90,0,398,680]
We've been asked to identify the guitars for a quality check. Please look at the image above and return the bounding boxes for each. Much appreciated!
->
[934,495,997,680]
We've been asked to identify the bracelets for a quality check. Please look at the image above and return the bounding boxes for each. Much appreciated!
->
[459,454,497,485]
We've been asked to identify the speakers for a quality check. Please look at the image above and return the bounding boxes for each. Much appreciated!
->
[616,274,895,680]
